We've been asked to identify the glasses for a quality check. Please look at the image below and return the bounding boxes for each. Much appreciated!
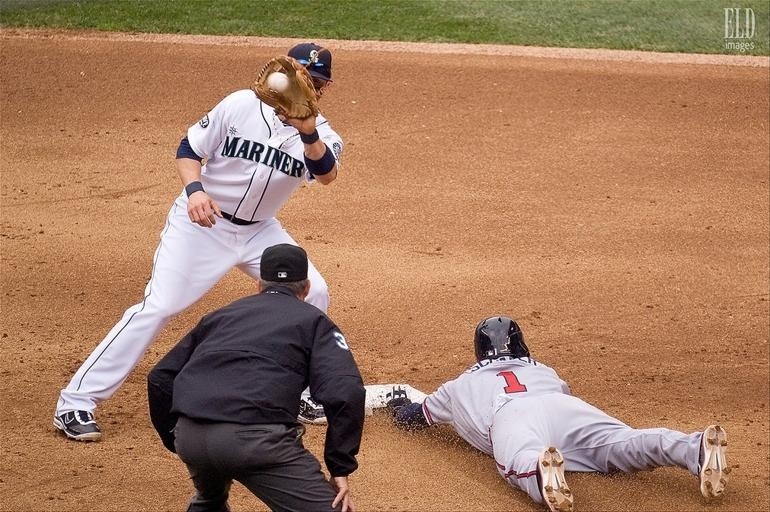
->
[298,59,331,78]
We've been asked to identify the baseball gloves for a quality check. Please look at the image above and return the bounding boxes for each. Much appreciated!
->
[395,403,427,428]
[254,54,319,119]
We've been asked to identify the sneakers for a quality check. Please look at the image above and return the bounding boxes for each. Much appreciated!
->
[537,447,575,512]
[697,425,729,499]
[53,410,102,441]
[296,397,329,425]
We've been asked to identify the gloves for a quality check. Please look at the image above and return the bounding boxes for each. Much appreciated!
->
[383,386,411,416]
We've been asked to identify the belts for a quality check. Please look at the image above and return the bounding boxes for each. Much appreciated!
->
[220,212,259,226]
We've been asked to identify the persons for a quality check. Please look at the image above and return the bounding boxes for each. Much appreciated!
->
[52,42,346,442]
[146,243,368,512]
[382,317,734,512]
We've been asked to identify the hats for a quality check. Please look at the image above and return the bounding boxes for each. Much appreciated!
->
[260,244,307,281]
[288,43,333,82]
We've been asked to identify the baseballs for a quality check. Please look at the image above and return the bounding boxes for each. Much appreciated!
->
[268,72,289,92]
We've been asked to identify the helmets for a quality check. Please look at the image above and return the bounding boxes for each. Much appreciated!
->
[474,317,530,358]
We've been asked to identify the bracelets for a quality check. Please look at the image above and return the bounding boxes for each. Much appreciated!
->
[184,182,205,197]
[299,130,320,146]
